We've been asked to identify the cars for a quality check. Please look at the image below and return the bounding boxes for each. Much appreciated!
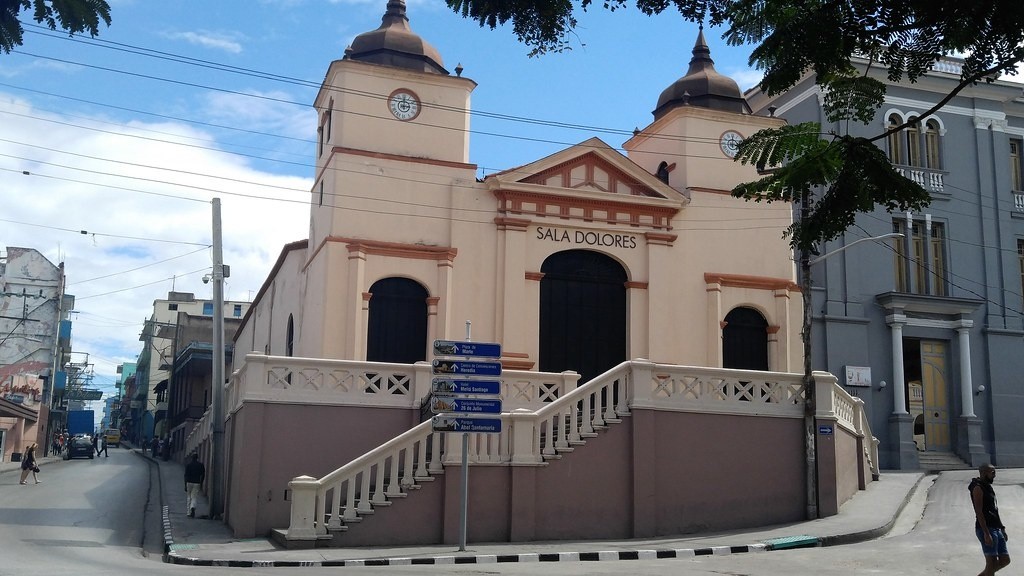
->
[68,433,95,460]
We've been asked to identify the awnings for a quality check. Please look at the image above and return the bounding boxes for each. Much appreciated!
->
[153,379,168,393]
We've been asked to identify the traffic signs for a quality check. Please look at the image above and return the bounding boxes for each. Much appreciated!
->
[431,416,502,434]
[432,359,502,377]
[431,396,503,414]
[431,378,502,396]
[434,340,501,359]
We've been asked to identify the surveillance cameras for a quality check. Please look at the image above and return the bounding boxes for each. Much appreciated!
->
[202,276,209,284]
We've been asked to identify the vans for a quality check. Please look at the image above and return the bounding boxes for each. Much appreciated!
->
[103,429,121,448]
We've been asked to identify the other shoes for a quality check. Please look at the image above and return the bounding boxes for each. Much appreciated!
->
[35,480,42,484]
[20,479,28,484]
[187,513,191,516]
[106,455,109,457]
[191,507,195,517]
[98,455,100,457]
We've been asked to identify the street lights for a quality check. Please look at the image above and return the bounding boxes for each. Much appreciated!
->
[803,232,906,519]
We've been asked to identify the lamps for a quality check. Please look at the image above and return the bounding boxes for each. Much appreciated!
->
[976,384,985,396]
[879,380,887,392]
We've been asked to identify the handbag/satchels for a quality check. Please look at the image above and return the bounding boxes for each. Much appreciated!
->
[33,466,40,472]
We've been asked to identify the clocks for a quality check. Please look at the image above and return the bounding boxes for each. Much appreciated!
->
[387,87,422,122]
[719,129,745,160]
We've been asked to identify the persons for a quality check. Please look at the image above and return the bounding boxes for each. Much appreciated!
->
[184,452,205,517]
[20,443,42,484]
[968,463,1011,576]
[51,428,69,456]
[142,435,173,458]
[93,434,110,458]
[120,429,138,445]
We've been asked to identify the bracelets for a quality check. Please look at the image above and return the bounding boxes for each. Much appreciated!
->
[1001,526,1005,530]
